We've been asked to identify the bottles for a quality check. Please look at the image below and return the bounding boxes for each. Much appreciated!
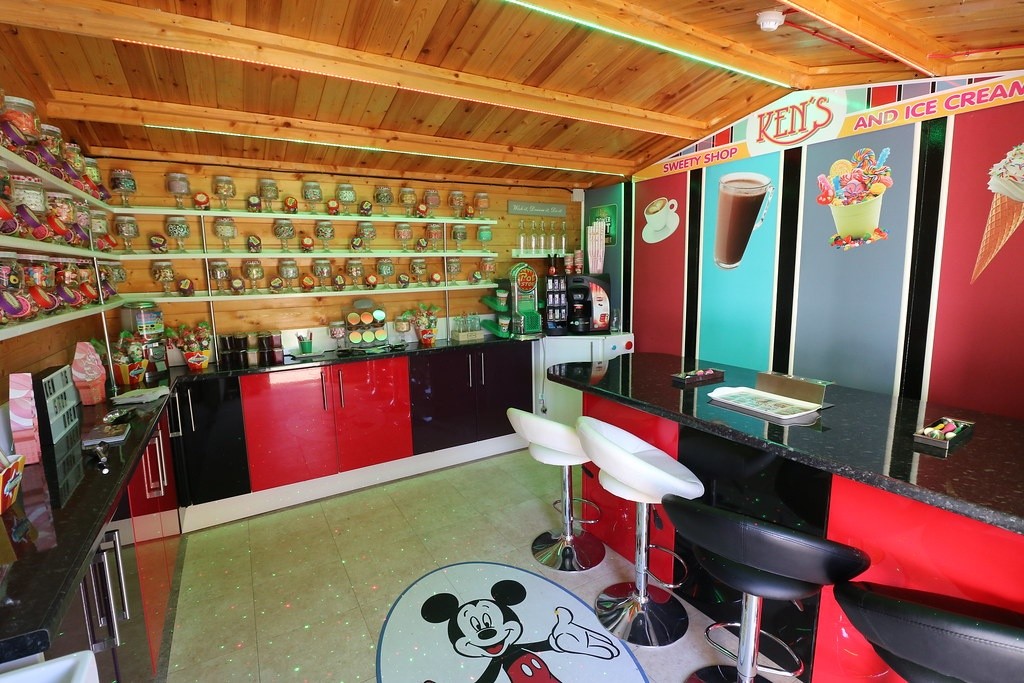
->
[140,345,161,390]
[517,218,569,256]
[453,310,481,333]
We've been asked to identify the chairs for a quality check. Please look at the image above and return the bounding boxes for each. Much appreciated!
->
[659,492,871,682]
[574,414,705,647]
[504,405,607,572]
[832,578,1024,683]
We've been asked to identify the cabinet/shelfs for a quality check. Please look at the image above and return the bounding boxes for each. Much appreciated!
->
[0,137,128,465]
[174,376,252,534]
[409,341,534,476]
[127,405,186,683]
[0,488,157,683]
[108,202,499,367]
[237,355,414,512]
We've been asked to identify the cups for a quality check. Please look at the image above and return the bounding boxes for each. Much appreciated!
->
[299,340,312,354]
[498,315,511,334]
[713,170,775,270]
[496,289,508,308]
[645,197,679,232]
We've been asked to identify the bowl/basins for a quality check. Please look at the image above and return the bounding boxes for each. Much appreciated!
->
[829,180,887,240]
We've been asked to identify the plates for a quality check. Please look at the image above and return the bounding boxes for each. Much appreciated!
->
[642,212,680,244]
